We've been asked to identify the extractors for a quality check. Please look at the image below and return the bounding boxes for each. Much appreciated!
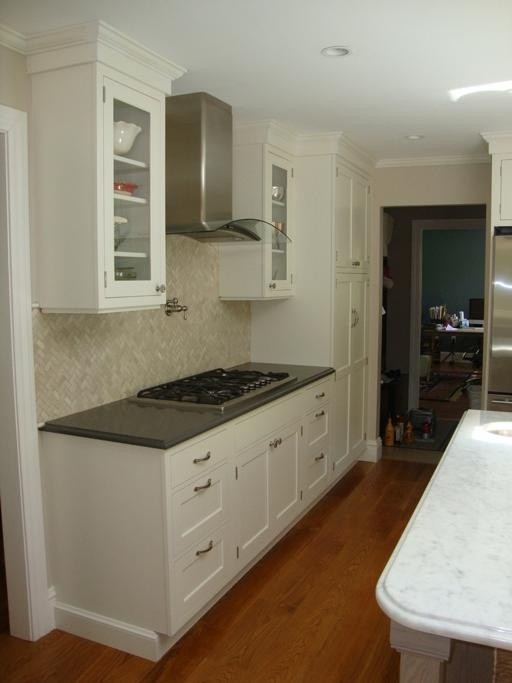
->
[166,89,292,250]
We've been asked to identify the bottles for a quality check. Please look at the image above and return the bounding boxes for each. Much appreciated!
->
[383,413,414,445]
[421,415,430,440]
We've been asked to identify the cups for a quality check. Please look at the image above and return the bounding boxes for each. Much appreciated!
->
[436,324,443,333]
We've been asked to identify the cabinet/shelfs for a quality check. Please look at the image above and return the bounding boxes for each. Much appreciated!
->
[31,59,168,314]
[252,153,369,484]
[231,386,305,573]
[38,420,233,637]
[219,141,297,301]
[304,372,334,507]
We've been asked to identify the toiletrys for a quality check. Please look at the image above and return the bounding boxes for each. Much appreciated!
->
[458,311,470,328]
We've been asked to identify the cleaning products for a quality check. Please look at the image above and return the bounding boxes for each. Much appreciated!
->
[384,414,416,447]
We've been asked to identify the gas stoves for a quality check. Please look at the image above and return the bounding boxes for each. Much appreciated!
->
[131,367,298,412]
